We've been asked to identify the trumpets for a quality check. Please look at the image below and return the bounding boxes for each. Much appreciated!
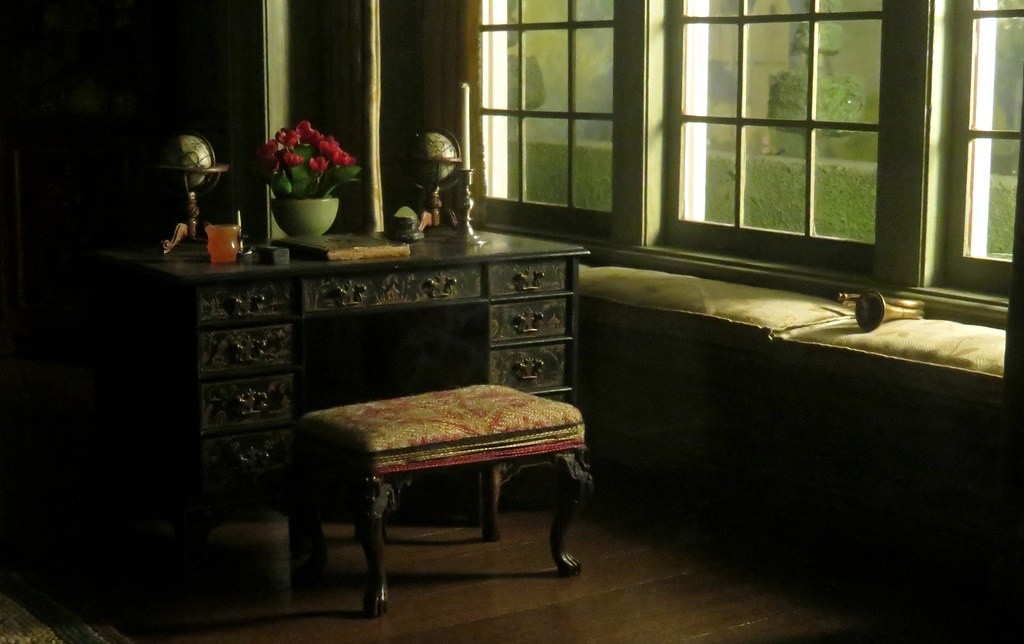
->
[837,287,927,334]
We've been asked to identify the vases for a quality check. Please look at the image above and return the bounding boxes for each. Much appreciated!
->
[269,198,339,237]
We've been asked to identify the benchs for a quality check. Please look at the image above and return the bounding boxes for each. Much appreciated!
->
[575,261,1009,552]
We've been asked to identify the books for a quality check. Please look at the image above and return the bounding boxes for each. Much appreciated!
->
[270,234,411,261]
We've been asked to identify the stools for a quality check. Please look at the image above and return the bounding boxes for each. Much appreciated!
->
[295,385,586,617]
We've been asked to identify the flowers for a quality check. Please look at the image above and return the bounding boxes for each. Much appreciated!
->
[256,120,363,201]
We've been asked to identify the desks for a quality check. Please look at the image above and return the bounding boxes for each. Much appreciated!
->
[82,229,592,560]
